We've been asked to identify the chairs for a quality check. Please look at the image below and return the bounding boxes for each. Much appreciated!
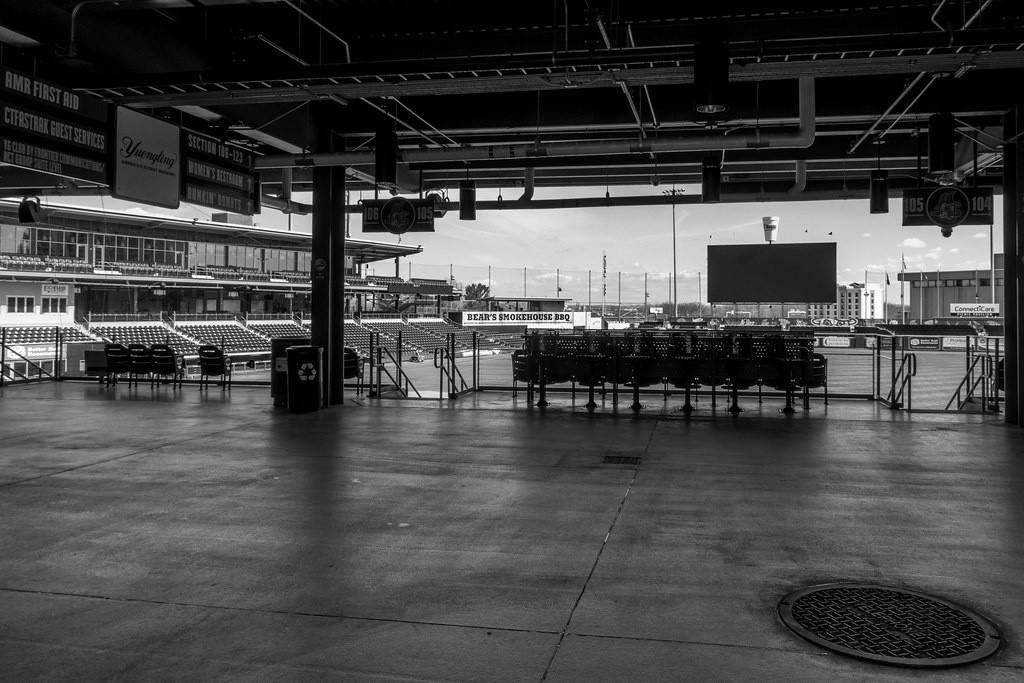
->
[176,324,232,345]
[97,326,176,342]
[0,326,95,344]
[232,319,827,415]
[104,343,137,388]
[0,252,451,287]
[198,345,232,393]
[184,346,197,355]
[150,344,184,390]
[127,343,161,390]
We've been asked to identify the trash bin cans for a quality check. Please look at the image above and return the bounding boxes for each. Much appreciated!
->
[286,345,320,412]
[270,335,312,407]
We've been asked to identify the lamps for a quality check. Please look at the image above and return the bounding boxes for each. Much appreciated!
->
[691,33,728,114]
[869,137,889,214]
[701,156,721,204]
[927,113,955,174]
[375,97,397,187]
[459,162,476,220]
[18,196,47,223]
